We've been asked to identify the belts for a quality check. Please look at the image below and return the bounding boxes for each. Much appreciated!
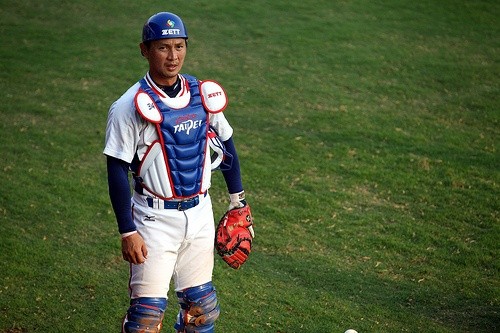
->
[146,190,207,211]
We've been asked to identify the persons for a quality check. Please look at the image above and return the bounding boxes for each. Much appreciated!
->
[99,10,254,333]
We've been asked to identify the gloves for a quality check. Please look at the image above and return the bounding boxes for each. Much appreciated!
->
[228,190,255,242]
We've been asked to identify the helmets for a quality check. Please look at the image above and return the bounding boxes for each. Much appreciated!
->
[142,12,188,41]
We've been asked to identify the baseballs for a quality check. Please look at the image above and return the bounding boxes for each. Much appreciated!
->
[345,329,358,333]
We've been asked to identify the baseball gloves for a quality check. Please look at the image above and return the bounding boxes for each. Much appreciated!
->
[214,201,254,270]
